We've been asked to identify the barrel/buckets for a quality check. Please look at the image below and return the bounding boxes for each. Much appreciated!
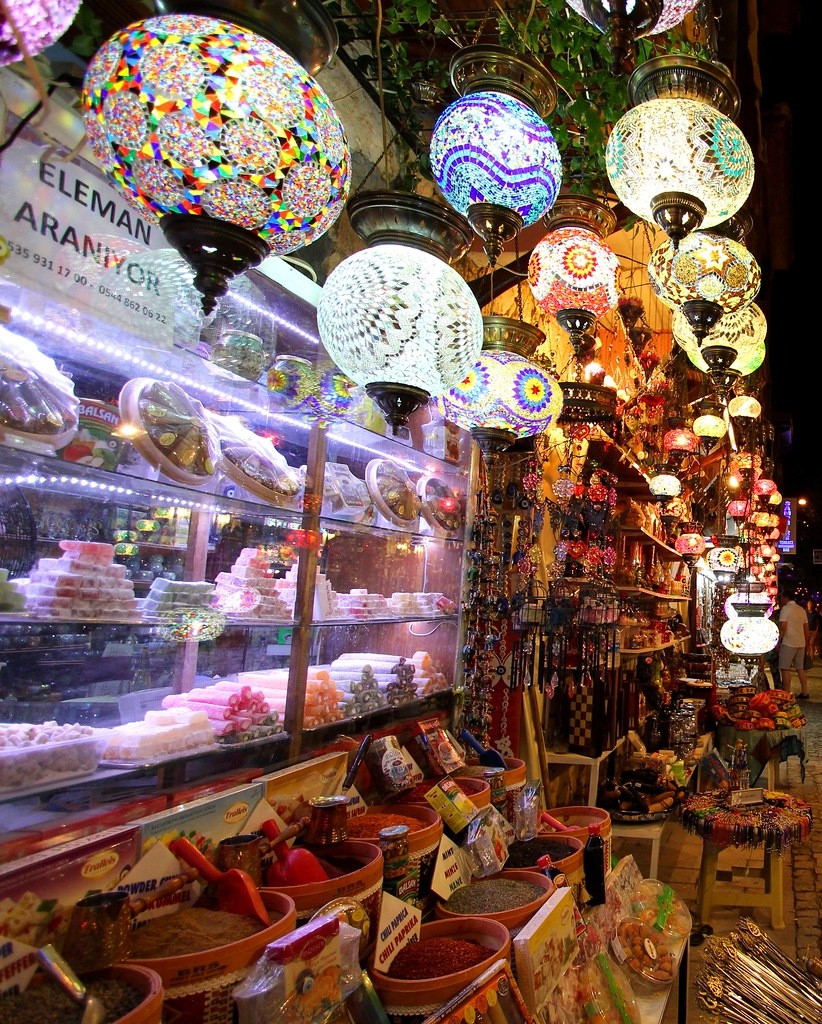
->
[0,750,613,1024]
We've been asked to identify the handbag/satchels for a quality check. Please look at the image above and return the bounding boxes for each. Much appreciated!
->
[803,646,811,670]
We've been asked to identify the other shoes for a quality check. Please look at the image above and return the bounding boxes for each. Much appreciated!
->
[795,692,809,699]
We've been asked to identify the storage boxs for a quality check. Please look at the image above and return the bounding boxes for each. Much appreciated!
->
[0,717,579,1024]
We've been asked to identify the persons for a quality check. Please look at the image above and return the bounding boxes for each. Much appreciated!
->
[768,586,822,701]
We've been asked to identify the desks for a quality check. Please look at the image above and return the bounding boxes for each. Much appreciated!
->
[723,726,806,788]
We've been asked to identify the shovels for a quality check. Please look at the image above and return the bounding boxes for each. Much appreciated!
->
[169,837,270,928]
[461,729,507,771]
[541,813,580,832]
[34,943,106,1024]
[308,734,373,808]
[262,818,330,887]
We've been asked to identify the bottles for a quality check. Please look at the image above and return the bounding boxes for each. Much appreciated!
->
[668,703,700,756]
[536,854,588,938]
[584,821,609,906]
[209,328,267,382]
[267,354,312,399]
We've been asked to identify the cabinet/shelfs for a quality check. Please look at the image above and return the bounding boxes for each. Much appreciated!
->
[541,715,712,878]
[0,278,476,862]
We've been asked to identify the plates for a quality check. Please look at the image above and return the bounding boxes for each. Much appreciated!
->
[1,377,462,535]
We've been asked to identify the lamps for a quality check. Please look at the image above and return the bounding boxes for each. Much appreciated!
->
[0,0,786,682]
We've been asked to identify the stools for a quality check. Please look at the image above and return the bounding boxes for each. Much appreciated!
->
[694,839,786,929]
